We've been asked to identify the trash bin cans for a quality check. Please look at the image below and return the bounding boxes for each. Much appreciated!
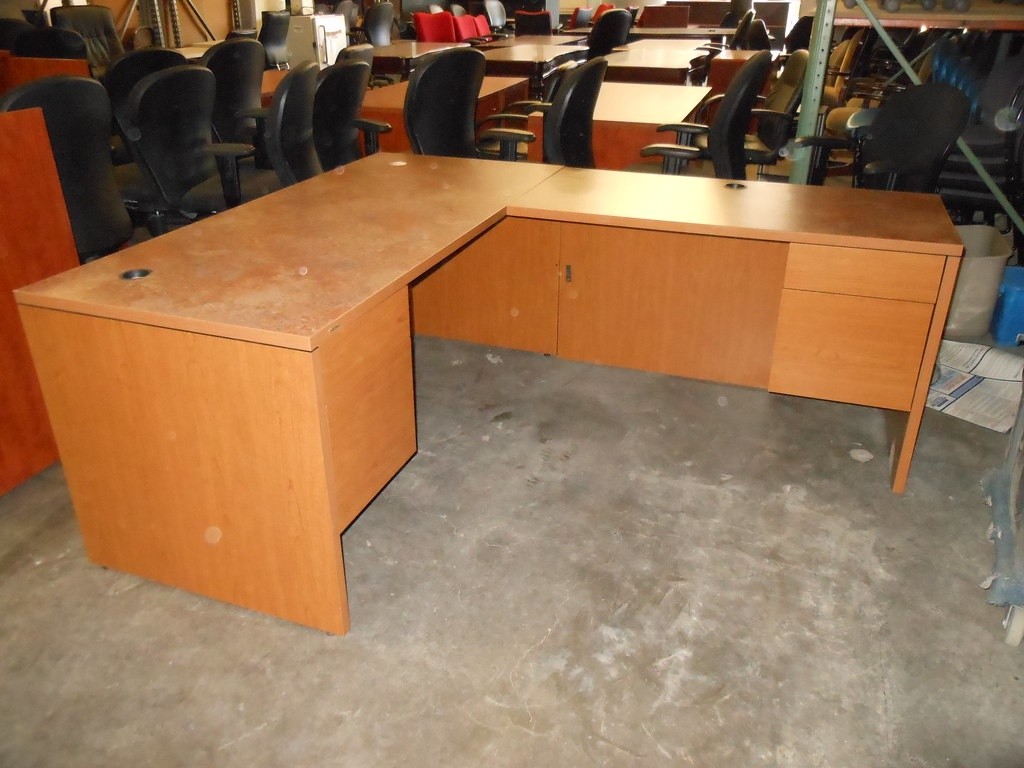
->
[990,265,1024,346]
[944,224,1013,340]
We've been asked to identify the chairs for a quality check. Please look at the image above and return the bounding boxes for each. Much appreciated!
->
[0,4,1023,265]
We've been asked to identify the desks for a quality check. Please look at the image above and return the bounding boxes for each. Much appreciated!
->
[0,0,959,631]
